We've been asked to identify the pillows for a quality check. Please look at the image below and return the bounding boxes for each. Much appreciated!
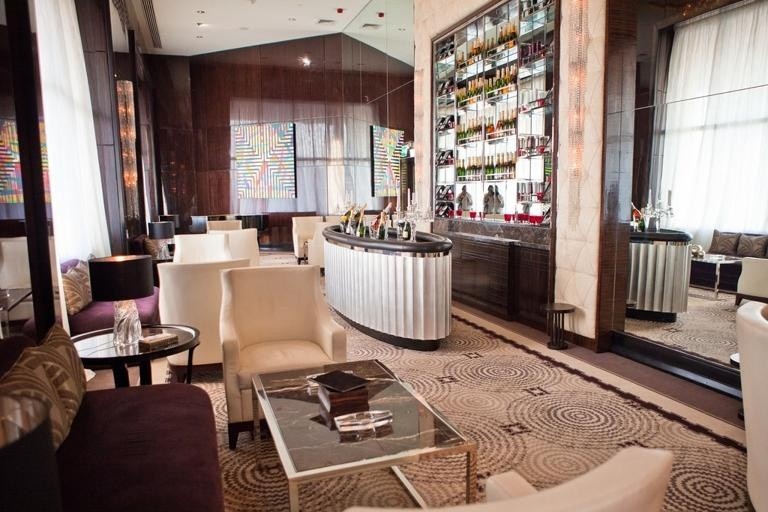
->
[75,260,90,276]
[1,346,72,450]
[66,266,94,305]
[735,234,768,258]
[60,267,88,315]
[708,229,740,257]
[24,319,89,427]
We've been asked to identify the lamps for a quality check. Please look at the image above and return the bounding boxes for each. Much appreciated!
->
[88,255,155,347]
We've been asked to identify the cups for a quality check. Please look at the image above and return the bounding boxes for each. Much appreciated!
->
[403,201,433,222]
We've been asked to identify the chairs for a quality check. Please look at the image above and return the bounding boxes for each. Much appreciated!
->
[734,257,768,304]
[127,213,262,269]
[733,302,768,512]
[217,263,347,450]
[291,214,378,265]
[156,256,249,384]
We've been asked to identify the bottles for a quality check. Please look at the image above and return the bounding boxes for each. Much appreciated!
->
[434,23,548,225]
[336,200,416,243]
[520,1,529,18]
[630,202,661,233]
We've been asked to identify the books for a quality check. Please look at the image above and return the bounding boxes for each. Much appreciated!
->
[306,369,372,394]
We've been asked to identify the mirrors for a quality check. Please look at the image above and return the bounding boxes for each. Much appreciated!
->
[607,0,768,401]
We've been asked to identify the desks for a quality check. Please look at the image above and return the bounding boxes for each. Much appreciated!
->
[626,223,693,324]
[71,323,203,388]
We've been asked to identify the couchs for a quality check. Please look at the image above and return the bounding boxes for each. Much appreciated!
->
[60,258,160,336]
[2,315,226,511]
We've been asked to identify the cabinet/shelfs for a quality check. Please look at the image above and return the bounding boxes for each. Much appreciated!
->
[431,0,556,228]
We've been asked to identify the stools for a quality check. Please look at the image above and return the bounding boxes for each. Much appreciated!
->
[539,302,577,350]
